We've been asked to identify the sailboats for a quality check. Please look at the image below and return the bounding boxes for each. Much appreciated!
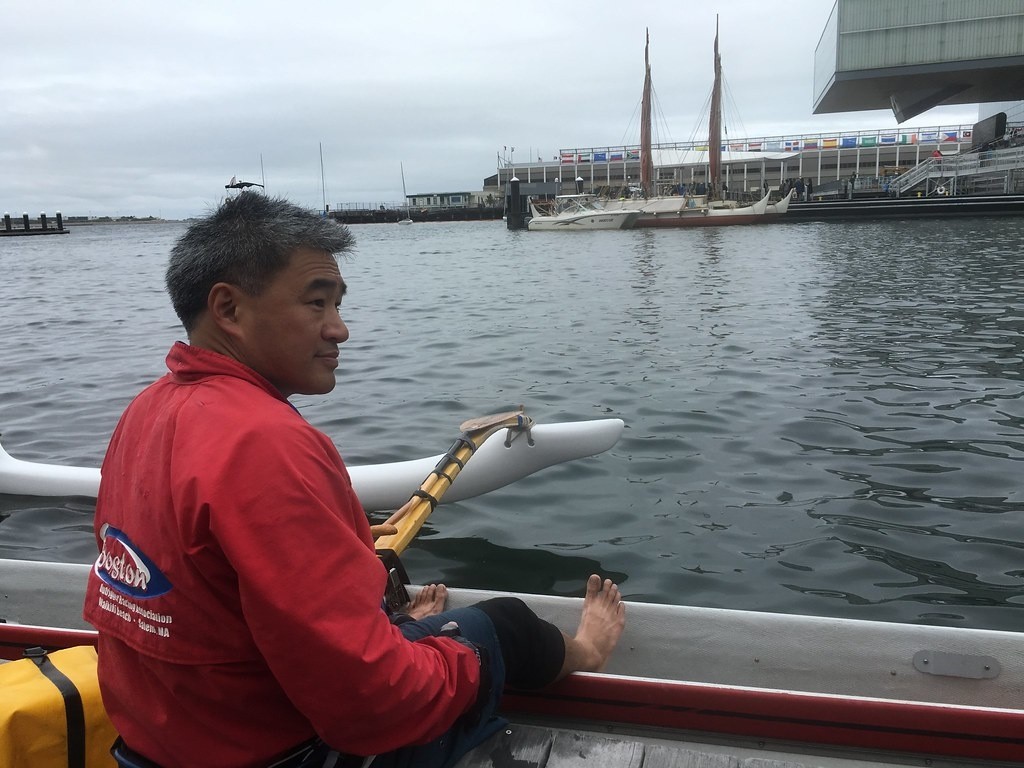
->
[319,141,337,224]
[398,161,413,226]
[527,14,794,231]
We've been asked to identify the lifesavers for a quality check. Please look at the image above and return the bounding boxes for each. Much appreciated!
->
[937,187,945,194]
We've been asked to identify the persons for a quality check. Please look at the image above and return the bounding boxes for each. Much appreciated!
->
[880,165,887,176]
[662,181,727,196]
[83,189,626,768]
[764,179,768,193]
[932,149,943,171]
[779,177,813,203]
[851,171,856,178]
[1003,127,1017,148]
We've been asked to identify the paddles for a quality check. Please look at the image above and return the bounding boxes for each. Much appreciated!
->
[373,410,536,565]
